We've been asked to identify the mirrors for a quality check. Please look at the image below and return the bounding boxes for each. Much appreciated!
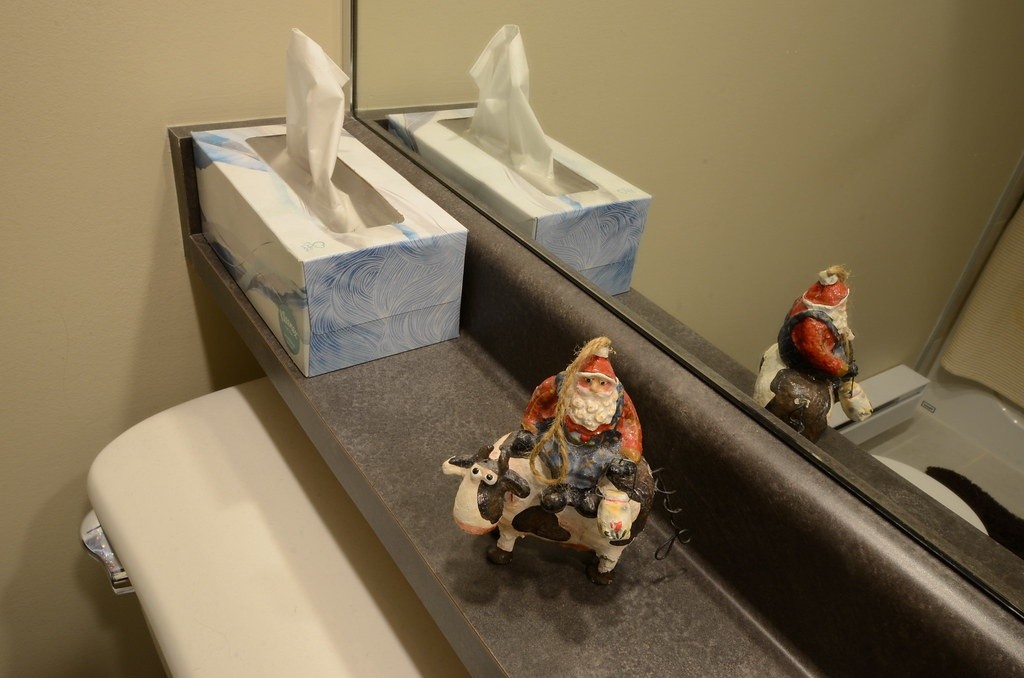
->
[341,0,1024,622]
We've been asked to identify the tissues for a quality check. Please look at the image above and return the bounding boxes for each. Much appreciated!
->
[384,20,656,302]
[189,21,473,380]
